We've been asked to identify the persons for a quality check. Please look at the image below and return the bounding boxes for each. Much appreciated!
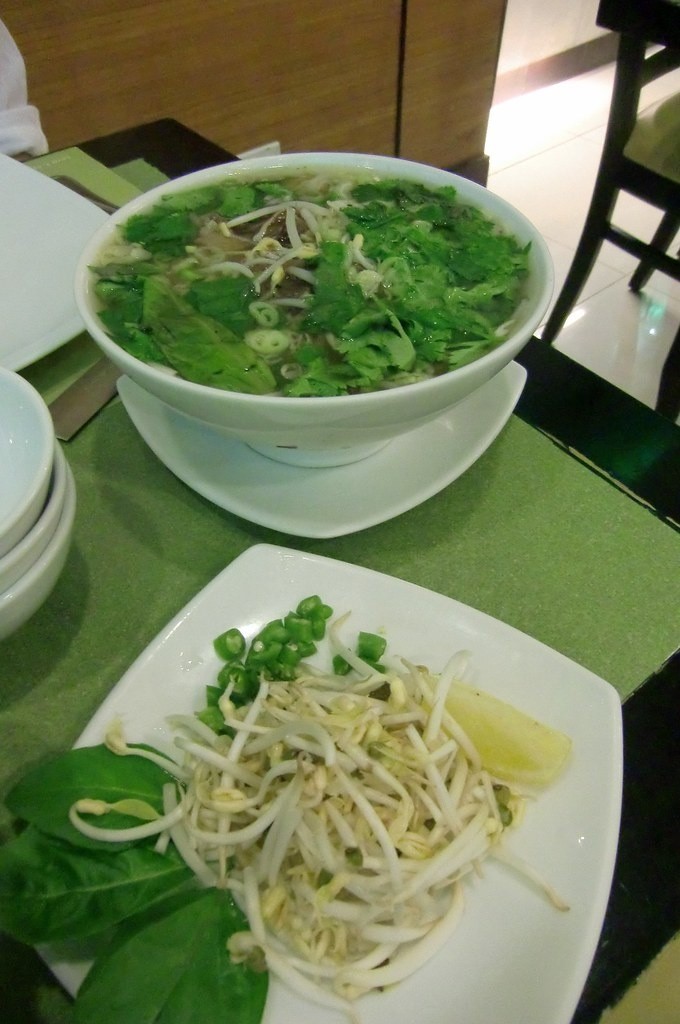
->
[0,20,50,165]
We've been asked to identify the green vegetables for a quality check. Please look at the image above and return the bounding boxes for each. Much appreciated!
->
[88,178,527,396]
[0,745,270,1024]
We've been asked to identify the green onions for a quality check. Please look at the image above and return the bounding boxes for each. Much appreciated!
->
[196,593,387,734]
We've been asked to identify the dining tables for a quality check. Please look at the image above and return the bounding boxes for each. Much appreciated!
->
[1,118,680,1024]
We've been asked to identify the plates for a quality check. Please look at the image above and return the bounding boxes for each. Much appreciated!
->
[114,359,529,539]
[25,543,624,1024]
[0,149,111,372]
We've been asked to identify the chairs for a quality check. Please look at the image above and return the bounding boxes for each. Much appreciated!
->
[540,1,679,421]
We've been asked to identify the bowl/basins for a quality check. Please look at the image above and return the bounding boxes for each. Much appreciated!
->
[0,364,77,643]
[74,151,554,468]
